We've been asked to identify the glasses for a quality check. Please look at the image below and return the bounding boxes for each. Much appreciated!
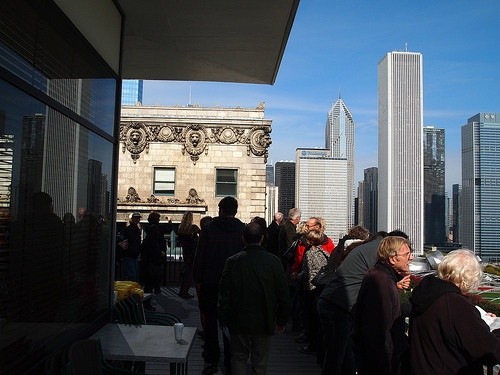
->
[397,252,410,259]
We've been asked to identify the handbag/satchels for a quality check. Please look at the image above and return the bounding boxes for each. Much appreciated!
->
[310,266,333,286]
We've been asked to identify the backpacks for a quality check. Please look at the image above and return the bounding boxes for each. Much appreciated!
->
[282,238,306,265]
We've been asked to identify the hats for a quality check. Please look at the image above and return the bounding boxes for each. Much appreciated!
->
[132,213,142,218]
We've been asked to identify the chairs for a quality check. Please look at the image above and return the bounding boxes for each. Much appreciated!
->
[44,293,188,375]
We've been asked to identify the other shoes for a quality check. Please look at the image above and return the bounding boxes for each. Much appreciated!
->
[295,331,306,342]
[179,290,194,298]
[299,343,315,354]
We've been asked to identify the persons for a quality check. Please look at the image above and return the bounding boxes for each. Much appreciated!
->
[217,221,290,375]
[12,193,111,320]
[114,196,500,375]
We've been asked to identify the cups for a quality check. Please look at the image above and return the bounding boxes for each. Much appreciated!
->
[174,323,184,340]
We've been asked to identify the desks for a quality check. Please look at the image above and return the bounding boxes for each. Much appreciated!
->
[88,323,198,375]
